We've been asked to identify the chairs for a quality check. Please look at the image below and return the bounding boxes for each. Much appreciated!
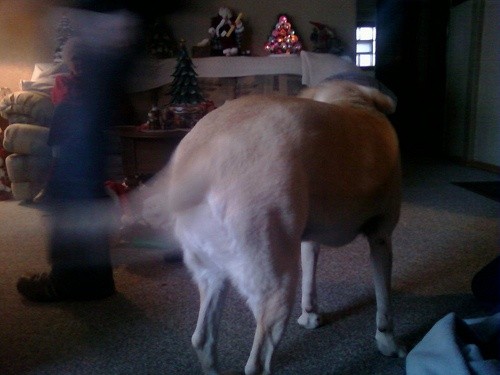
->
[2,83,124,201]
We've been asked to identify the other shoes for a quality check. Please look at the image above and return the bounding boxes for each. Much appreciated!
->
[15,272,115,303]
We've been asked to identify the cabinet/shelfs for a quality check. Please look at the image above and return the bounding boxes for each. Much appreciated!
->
[117,53,314,175]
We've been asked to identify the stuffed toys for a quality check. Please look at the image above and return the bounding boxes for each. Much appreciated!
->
[209,4,247,57]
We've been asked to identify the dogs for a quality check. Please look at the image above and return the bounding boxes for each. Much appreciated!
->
[33,78,410,374]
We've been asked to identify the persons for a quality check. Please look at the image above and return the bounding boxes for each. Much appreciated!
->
[15,33,119,303]
